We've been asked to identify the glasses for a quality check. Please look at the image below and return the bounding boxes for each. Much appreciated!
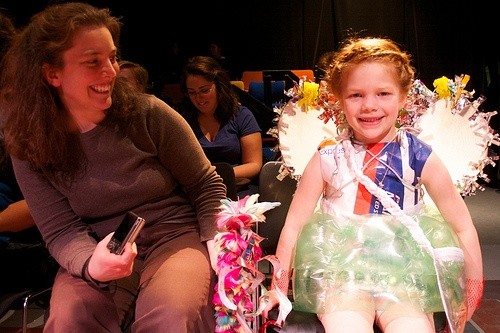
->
[185,85,215,97]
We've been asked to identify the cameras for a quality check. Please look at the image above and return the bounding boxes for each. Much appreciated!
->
[106,211,145,255]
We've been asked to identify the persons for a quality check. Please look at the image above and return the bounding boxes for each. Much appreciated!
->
[117,58,148,95]
[1,137,60,298]
[0,2,226,333]
[261,39,482,332]
[180,55,263,200]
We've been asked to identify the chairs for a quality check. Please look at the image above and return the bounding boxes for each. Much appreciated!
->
[250,81,286,145]
[0,161,448,333]
